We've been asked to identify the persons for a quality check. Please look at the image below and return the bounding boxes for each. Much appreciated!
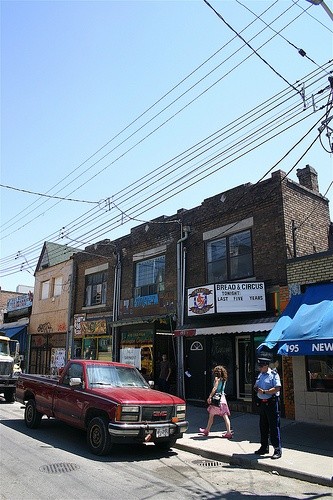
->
[253,356,282,459]
[157,353,172,392]
[198,366,232,438]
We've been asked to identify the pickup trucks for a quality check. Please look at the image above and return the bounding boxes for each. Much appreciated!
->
[15,359,190,457]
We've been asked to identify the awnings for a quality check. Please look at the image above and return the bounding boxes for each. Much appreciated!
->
[256,280,333,357]
[174,315,280,337]
[0,326,27,352]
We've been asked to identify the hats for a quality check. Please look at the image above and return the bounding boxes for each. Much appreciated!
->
[257,358,271,365]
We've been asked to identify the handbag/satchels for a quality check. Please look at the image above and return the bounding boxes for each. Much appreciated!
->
[210,395,221,407]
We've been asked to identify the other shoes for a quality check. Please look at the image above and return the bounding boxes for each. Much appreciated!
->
[255,448,268,454]
[199,427,209,436]
[271,451,281,458]
[222,433,232,438]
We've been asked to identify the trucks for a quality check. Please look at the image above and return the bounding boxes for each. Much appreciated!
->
[0,332,24,403]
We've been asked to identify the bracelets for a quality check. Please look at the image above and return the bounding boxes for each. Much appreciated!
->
[263,390,265,394]
